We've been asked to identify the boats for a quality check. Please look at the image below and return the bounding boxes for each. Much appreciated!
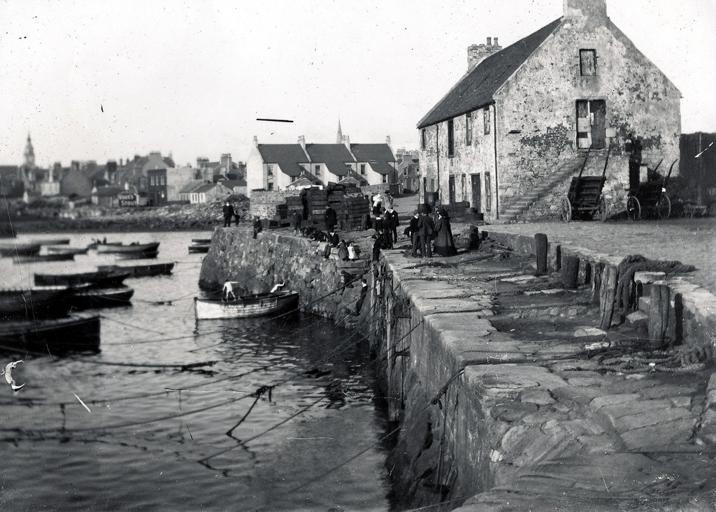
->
[191,237,211,243]
[191,290,298,320]
[0,223,178,367]
[188,243,209,249]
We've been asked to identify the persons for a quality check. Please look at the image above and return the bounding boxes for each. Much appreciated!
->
[324,379,347,410]
[222,278,241,303]
[252,216,263,239]
[631,139,643,164]
[222,199,235,227]
[234,210,240,228]
[292,188,459,312]
[270,280,285,293]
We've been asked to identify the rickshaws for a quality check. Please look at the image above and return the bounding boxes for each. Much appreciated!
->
[556,142,613,223]
[625,157,681,220]
